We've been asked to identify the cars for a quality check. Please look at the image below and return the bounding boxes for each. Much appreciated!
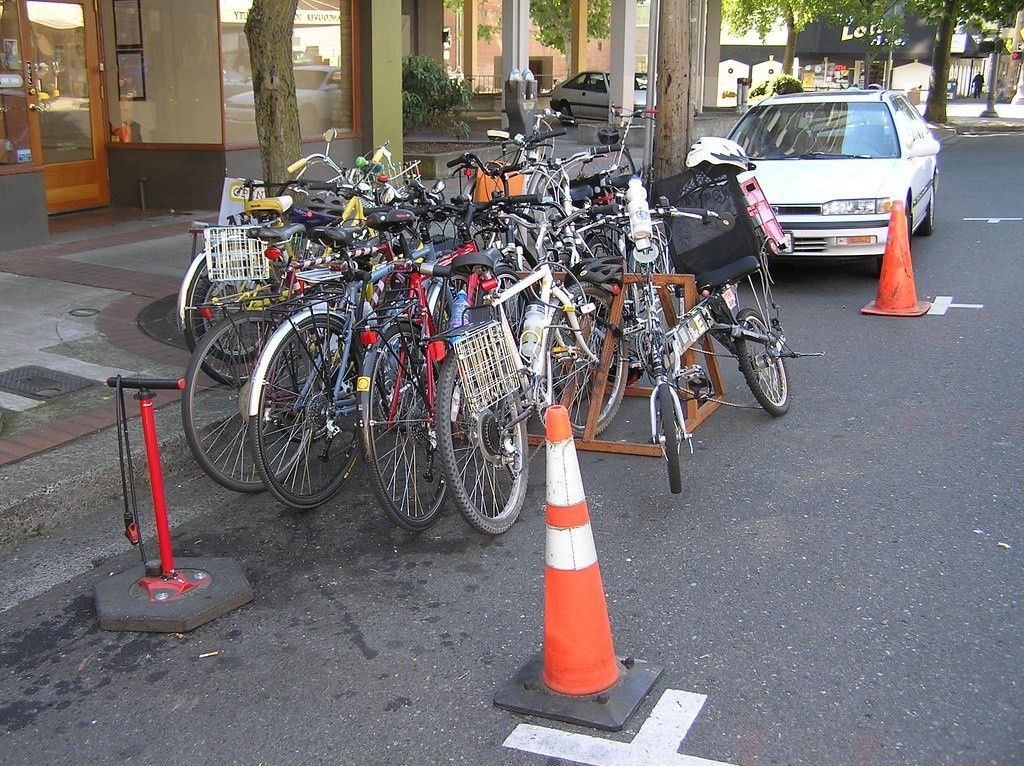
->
[293,50,311,67]
[550,70,649,126]
[726,89,940,276]
[224,65,343,135]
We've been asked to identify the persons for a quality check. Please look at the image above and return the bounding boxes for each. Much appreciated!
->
[972,71,984,99]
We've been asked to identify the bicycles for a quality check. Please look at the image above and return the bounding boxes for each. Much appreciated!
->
[179,108,827,534]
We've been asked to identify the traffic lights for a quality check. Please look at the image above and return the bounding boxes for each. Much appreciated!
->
[1012,52,1022,60]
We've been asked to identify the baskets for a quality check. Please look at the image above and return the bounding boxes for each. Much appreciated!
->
[326,159,422,195]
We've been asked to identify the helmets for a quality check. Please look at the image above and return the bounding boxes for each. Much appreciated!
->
[686,137,748,172]
[287,193,348,228]
[564,254,624,295]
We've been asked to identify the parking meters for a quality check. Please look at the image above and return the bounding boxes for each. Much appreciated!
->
[505,68,539,138]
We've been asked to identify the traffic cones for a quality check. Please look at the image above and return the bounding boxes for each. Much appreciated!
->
[861,200,930,315]
[492,404,664,730]
